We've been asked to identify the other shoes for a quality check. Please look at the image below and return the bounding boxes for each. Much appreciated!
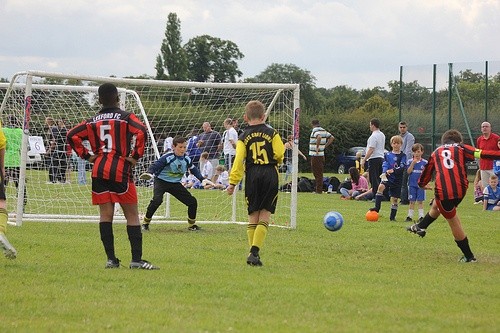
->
[372,199,376,202]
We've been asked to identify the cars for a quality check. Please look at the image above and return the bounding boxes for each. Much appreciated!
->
[335,147,388,174]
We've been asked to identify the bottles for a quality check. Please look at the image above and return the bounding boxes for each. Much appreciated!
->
[328,184,333,192]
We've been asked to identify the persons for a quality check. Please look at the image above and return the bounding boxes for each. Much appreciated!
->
[68,83,160,271]
[226,99,291,265]
[0,119,18,261]
[139,136,216,231]
[309,119,334,194]
[370,135,407,222]
[474,122,500,212]
[131,117,310,193]
[405,128,500,264]
[40,112,91,186]
[339,153,430,204]
[1,115,26,212]
[360,118,385,202]
[395,121,415,206]
[406,143,429,223]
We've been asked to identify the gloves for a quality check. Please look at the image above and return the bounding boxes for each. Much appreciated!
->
[201,179,215,188]
[139,172,153,180]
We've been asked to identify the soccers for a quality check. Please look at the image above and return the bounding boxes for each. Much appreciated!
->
[366,210,379,222]
[323,211,344,232]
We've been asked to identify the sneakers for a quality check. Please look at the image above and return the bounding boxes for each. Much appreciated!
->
[417,217,423,222]
[140,223,150,232]
[458,256,478,263]
[404,216,413,222]
[369,207,380,213]
[187,225,200,231]
[389,216,396,221]
[405,223,426,238]
[105,257,122,269]
[130,261,161,271]
[246,251,264,267]
